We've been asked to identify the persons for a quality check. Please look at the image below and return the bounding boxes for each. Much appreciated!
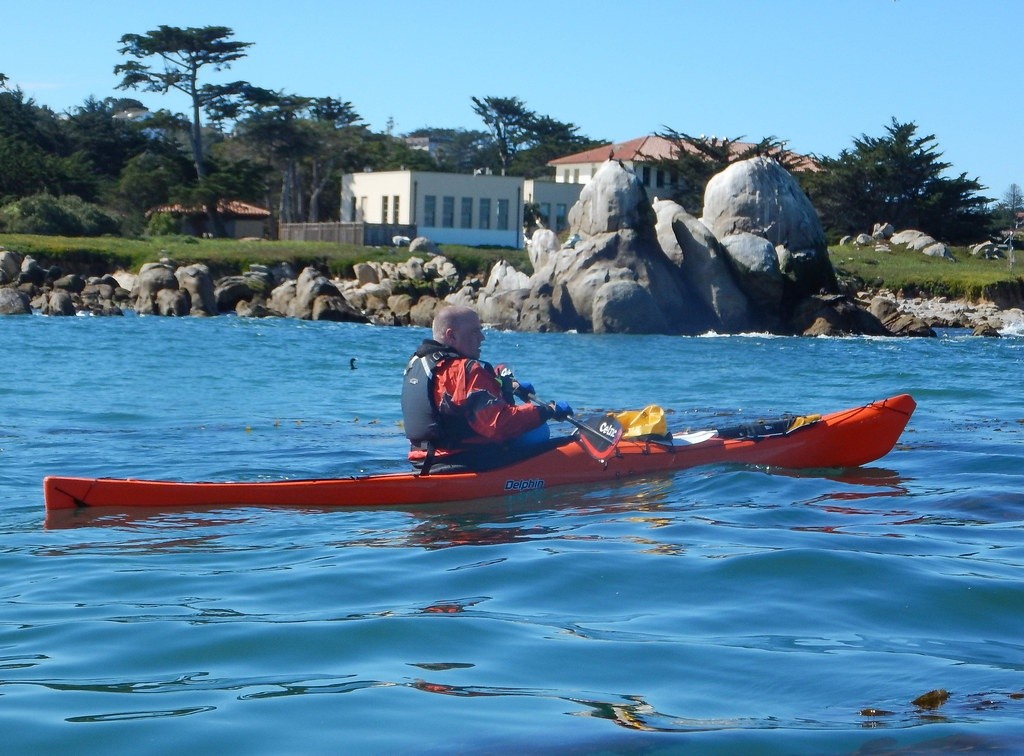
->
[400,305,575,476]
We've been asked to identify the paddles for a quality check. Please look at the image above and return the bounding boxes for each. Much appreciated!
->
[494,361,624,462]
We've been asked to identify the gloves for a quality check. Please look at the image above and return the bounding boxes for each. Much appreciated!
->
[513,382,536,404]
[549,399,574,422]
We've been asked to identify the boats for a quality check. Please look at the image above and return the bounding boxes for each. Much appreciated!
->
[45,393,916,529]
